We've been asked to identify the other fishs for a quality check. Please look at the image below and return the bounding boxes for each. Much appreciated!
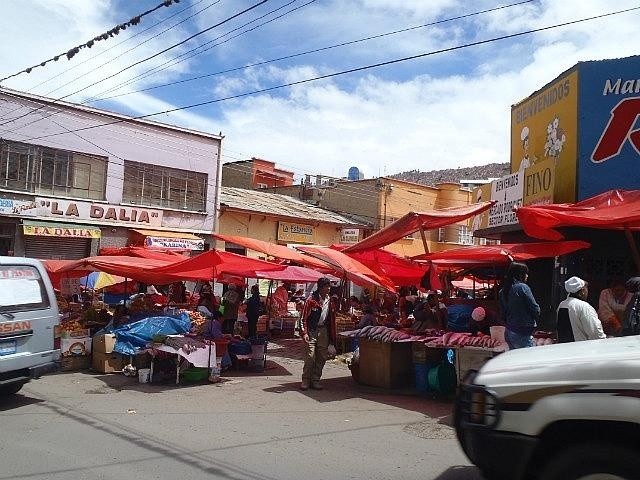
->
[424,330,503,347]
[347,325,425,344]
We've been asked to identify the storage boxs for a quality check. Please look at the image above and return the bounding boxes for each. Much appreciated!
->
[59,333,123,375]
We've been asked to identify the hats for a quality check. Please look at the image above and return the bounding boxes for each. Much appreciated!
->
[564,276,586,293]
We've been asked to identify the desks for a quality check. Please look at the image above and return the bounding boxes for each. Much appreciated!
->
[149,340,216,383]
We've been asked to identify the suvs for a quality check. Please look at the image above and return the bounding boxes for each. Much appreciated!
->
[453,333,639,480]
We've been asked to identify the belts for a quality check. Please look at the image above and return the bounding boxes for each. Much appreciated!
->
[318,325,325,328]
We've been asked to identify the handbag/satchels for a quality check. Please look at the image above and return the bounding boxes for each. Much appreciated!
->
[627,294,640,334]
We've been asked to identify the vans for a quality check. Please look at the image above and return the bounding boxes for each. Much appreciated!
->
[0,255,63,396]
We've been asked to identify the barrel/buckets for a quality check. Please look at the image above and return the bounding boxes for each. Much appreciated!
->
[415,365,428,391]
[250,360,265,373]
[489,325,505,340]
[252,345,264,360]
[428,362,456,392]
[138,368,150,383]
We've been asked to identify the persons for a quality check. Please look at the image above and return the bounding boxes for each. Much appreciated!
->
[553,275,607,343]
[597,275,633,337]
[274,282,291,305]
[130,277,261,337]
[494,261,543,351]
[623,277,640,337]
[299,278,337,390]
[351,285,475,337]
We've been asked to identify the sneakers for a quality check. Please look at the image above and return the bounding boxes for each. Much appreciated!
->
[300,382,310,390]
[310,382,323,390]
